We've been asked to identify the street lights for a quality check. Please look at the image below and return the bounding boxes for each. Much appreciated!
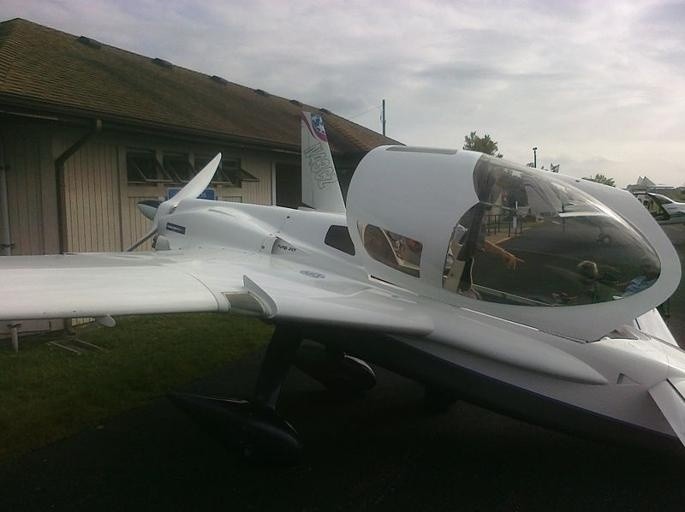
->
[532,147,538,168]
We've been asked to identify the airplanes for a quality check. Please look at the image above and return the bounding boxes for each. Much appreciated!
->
[1,109,684,446]
[512,185,685,246]
